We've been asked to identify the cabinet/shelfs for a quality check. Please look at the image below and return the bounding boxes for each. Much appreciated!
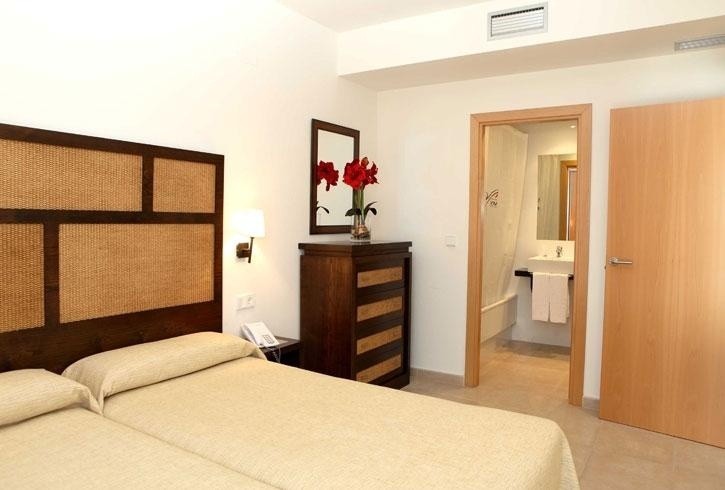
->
[298,241,412,391]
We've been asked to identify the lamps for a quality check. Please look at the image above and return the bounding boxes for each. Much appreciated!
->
[235,208,264,264]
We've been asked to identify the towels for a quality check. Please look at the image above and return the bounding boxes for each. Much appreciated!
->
[532,271,570,324]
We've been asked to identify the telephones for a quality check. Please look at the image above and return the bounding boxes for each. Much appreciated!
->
[240,321,279,348]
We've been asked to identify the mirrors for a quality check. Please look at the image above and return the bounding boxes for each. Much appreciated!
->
[309,118,361,234]
[536,152,578,242]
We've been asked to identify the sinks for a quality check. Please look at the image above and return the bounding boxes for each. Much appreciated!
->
[527,255,574,274]
[481,294,517,346]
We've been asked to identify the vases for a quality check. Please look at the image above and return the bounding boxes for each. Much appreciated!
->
[349,214,371,242]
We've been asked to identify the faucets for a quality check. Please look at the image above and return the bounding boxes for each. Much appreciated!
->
[557,246,563,257]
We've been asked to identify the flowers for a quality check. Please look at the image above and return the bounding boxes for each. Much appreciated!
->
[316,159,340,191]
[342,156,377,223]
